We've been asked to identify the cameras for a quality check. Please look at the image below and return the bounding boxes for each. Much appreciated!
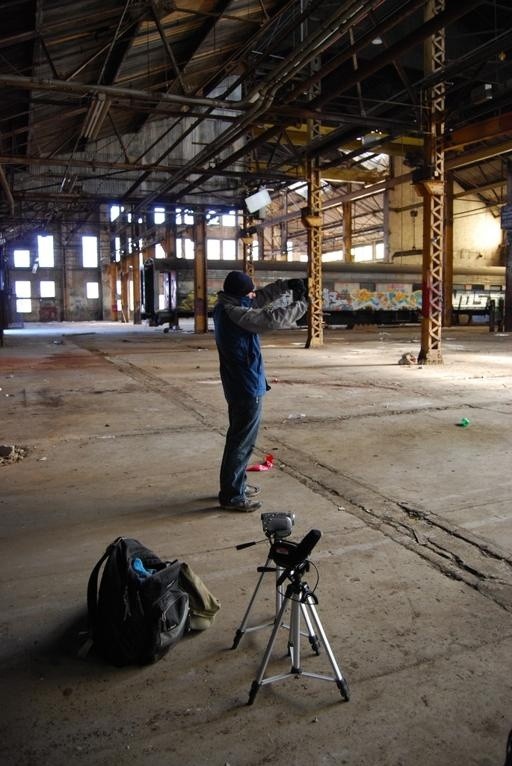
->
[260,512,295,538]
[270,528,322,581]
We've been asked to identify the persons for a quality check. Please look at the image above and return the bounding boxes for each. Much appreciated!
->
[212,271,310,512]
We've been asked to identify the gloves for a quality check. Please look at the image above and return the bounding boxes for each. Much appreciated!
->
[288,279,307,301]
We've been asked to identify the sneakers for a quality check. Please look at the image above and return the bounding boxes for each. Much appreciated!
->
[219,486,262,512]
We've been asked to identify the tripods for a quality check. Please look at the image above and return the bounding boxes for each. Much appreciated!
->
[231,539,322,656]
[247,577,352,706]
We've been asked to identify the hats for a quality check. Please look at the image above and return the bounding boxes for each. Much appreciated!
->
[224,271,254,297]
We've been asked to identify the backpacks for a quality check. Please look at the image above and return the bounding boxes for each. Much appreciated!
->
[87,535,221,668]
